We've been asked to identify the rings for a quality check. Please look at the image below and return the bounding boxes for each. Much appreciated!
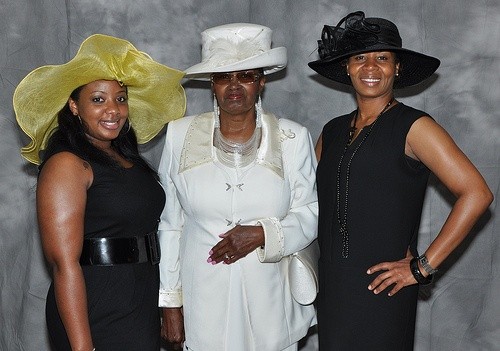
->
[225,252,236,263]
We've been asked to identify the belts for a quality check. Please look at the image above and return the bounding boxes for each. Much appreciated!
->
[79,231,161,267]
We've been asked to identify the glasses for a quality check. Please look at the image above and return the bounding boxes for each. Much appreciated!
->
[210,71,263,84]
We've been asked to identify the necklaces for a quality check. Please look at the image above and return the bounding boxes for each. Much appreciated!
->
[336,96,395,259]
[214,122,261,169]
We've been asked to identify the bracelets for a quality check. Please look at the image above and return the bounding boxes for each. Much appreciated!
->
[410,254,439,286]
[92,347,95,351]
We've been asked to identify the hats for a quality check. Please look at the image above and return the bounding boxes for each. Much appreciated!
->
[181,22,288,82]
[308,10,441,89]
[13,33,187,165]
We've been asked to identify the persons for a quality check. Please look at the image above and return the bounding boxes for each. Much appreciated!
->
[306,11,494,351]
[13,33,187,351]
[156,23,319,351]
